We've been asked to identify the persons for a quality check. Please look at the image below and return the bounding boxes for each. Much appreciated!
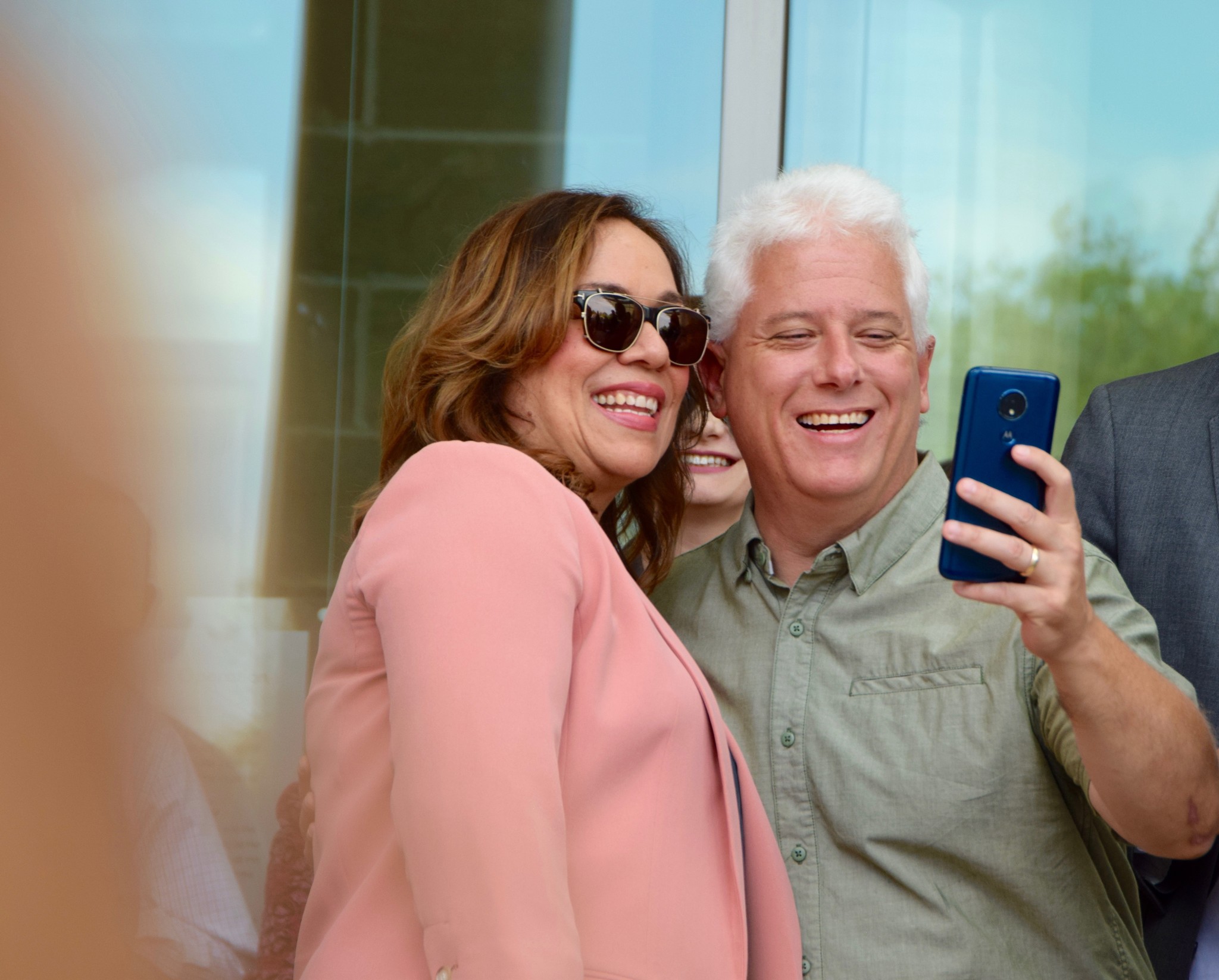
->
[294,192,802,980]
[299,162,1219,980]
[85,479,276,929]
[1055,351,1219,980]
[630,293,751,584]
[132,708,258,980]
[0,71,144,980]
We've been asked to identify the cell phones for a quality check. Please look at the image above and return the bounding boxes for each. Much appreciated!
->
[938,367,1063,581]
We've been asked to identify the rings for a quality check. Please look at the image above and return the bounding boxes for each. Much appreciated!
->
[1020,545,1038,575]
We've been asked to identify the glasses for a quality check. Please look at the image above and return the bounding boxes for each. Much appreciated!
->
[572,289,712,366]
